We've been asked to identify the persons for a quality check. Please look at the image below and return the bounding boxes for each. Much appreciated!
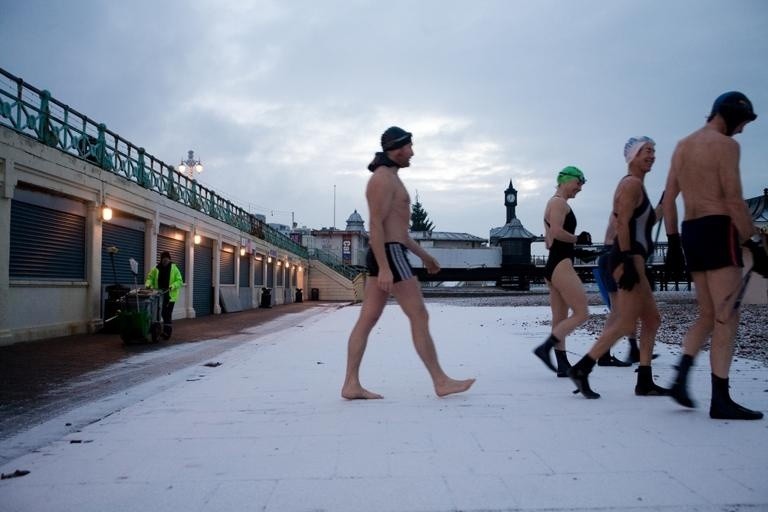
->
[529,165,598,377]
[145,250,184,341]
[659,88,768,419]
[338,125,477,401]
[564,134,670,398]
[598,212,659,368]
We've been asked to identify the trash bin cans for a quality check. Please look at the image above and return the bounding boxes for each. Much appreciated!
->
[120,289,164,345]
[311,288,319,301]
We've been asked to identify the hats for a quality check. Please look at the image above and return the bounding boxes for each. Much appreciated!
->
[557,166,586,185]
[710,89,758,136]
[381,126,413,151]
[623,135,656,165]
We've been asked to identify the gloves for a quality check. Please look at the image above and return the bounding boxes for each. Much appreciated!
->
[617,251,641,291]
[576,230,592,244]
[664,233,686,272]
[740,232,767,279]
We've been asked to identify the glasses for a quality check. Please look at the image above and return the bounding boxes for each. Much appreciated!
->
[560,171,586,184]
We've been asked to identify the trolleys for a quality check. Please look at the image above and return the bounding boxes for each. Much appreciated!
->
[115,283,172,347]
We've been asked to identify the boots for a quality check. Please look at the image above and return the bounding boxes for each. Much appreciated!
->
[669,354,697,409]
[633,366,669,396]
[566,354,600,399]
[707,373,764,419]
[531,332,572,379]
[597,339,660,367]
